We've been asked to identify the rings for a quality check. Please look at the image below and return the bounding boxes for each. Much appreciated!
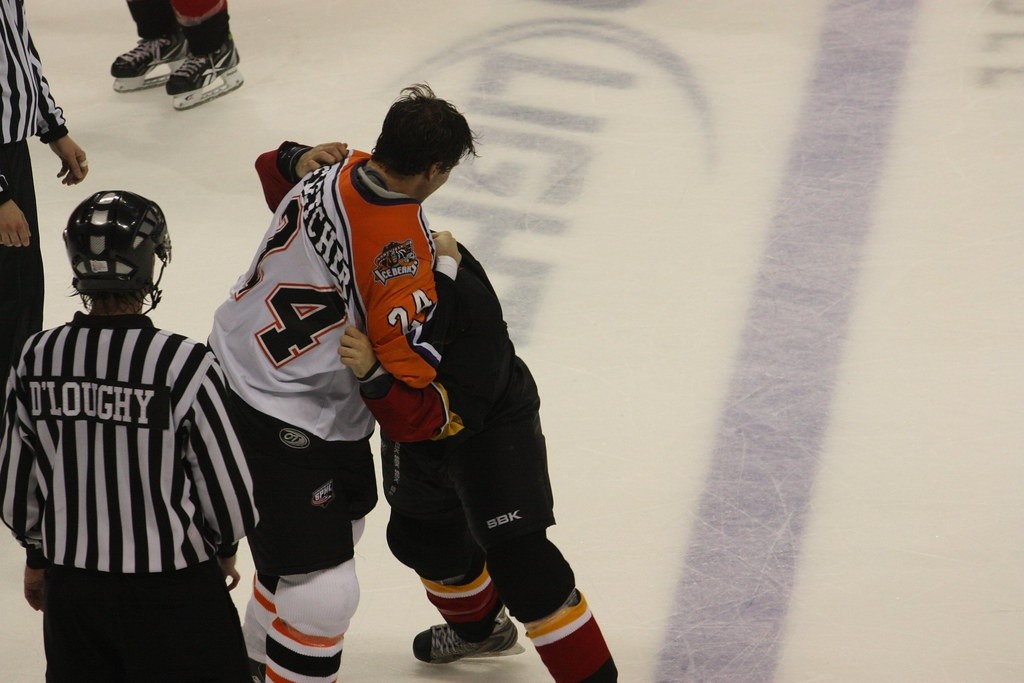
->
[80,159,88,169]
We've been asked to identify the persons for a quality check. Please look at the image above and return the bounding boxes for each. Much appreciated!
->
[110,0,242,112]
[0,187,260,683]
[198,79,478,683]
[254,138,620,683]
[1,1,89,433]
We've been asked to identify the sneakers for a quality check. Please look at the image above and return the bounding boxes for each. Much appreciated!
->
[110,41,167,92]
[166,30,244,111]
[412,605,518,666]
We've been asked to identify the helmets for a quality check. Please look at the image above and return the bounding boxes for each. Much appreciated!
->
[62,190,172,325]
